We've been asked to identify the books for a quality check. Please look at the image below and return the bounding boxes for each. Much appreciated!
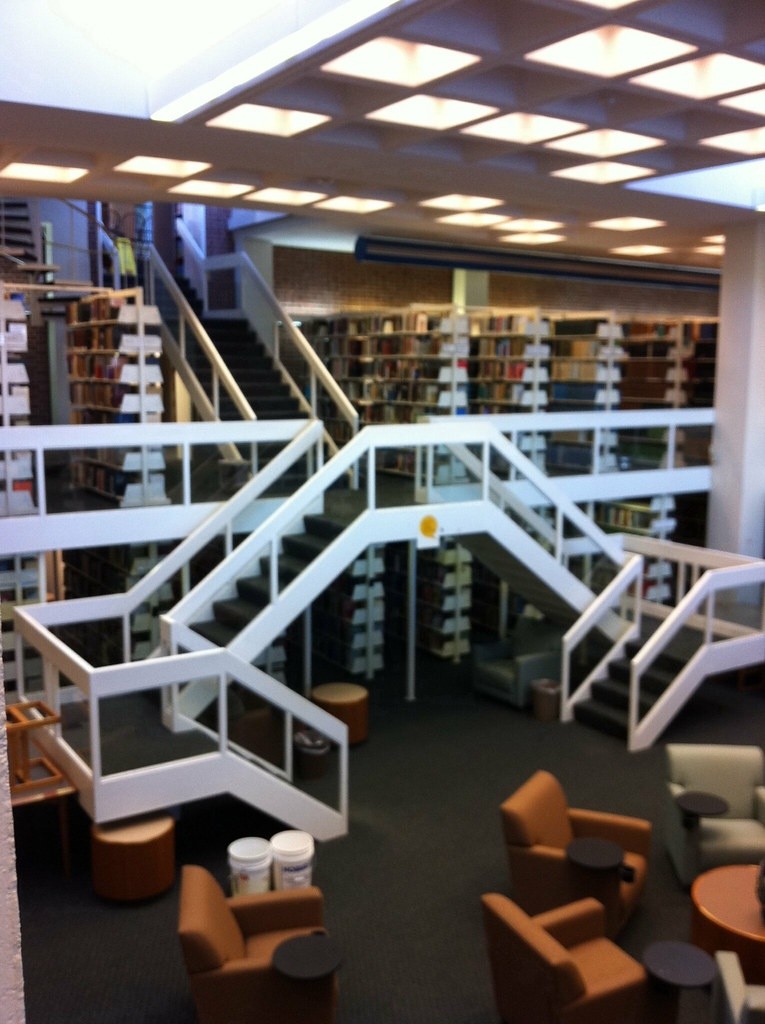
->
[301,313,720,474]
[67,298,137,495]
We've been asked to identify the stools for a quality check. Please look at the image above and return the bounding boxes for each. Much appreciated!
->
[92,814,174,903]
[311,683,368,745]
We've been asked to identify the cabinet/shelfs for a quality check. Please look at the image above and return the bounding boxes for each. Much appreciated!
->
[0,298,40,517]
[592,494,677,602]
[469,306,552,475]
[316,546,383,681]
[298,311,449,477]
[386,540,468,663]
[66,286,167,506]
[548,317,628,470]
[618,319,693,465]
[61,545,173,660]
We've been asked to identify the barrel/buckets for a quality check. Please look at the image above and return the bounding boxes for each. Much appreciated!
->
[227,837,273,896]
[271,830,315,889]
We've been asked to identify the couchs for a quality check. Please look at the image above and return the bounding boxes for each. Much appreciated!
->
[481,892,648,1024]
[472,616,562,709]
[177,865,339,1024]
[664,744,765,885]
[499,769,652,936]
[711,950,765,1024]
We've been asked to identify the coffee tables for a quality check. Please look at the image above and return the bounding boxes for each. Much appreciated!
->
[690,864,765,963]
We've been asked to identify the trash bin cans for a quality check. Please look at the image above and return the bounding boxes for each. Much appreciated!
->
[530,678,561,722]
[294,729,331,777]
